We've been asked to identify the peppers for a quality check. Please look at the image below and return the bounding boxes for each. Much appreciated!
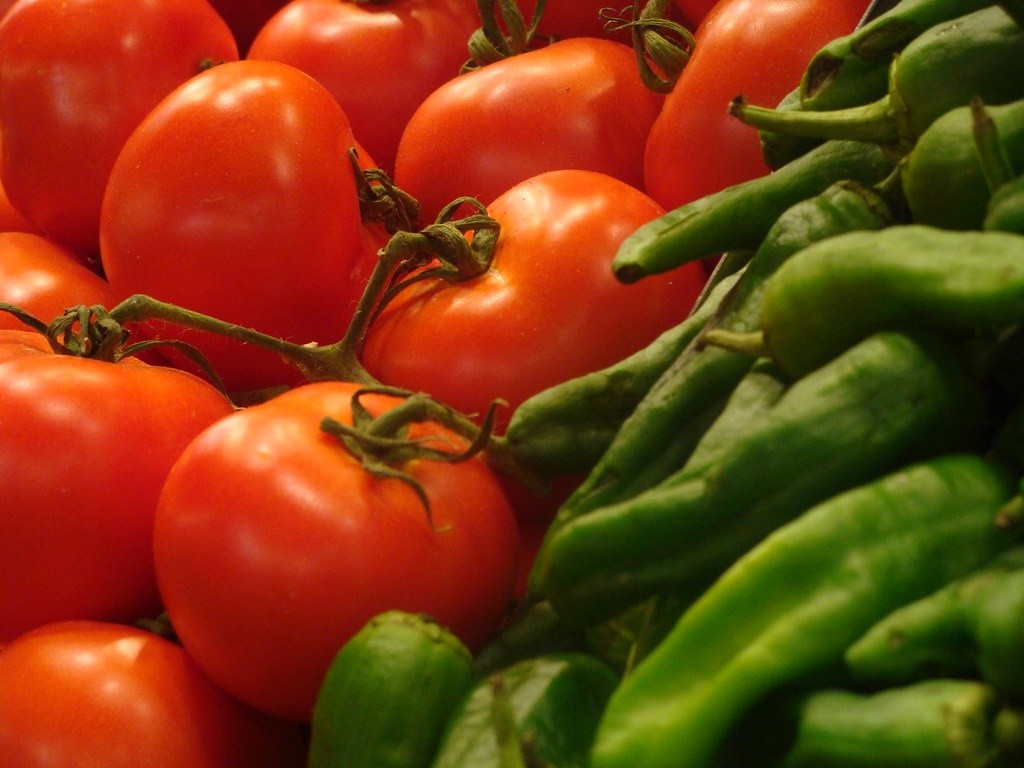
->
[306,0,1024,768]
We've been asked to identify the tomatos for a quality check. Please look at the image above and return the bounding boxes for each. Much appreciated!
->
[0,0,872,768]
